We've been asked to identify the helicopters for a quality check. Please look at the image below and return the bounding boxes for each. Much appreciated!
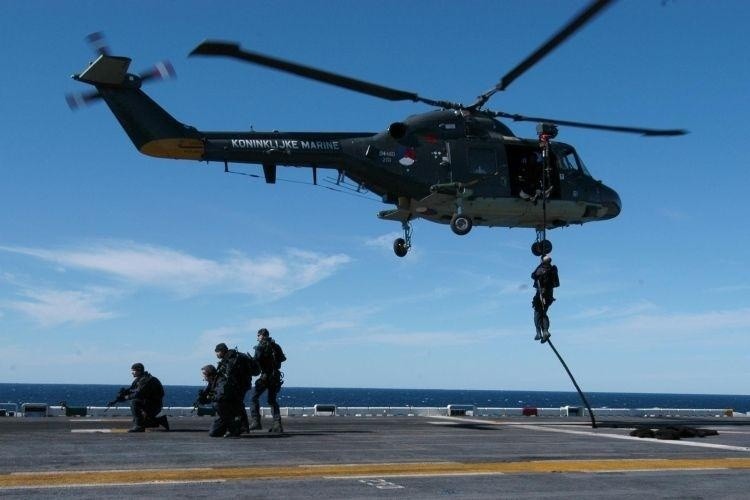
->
[62,1,690,260]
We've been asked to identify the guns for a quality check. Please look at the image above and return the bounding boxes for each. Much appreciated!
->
[216,359,226,374]
[104,386,132,412]
[190,382,213,414]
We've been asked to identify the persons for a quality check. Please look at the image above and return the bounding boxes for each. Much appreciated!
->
[246,328,287,433]
[122,362,171,433]
[214,342,252,434]
[191,366,241,438]
[531,255,560,343]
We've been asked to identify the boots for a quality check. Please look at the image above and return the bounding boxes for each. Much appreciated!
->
[269,420,283,433]
[224,414,262,439]
[535,328,551,343]
[529,185,553,206]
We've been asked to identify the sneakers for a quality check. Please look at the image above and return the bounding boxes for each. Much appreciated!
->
[159,415,169,432]
[127,425,145,432]
